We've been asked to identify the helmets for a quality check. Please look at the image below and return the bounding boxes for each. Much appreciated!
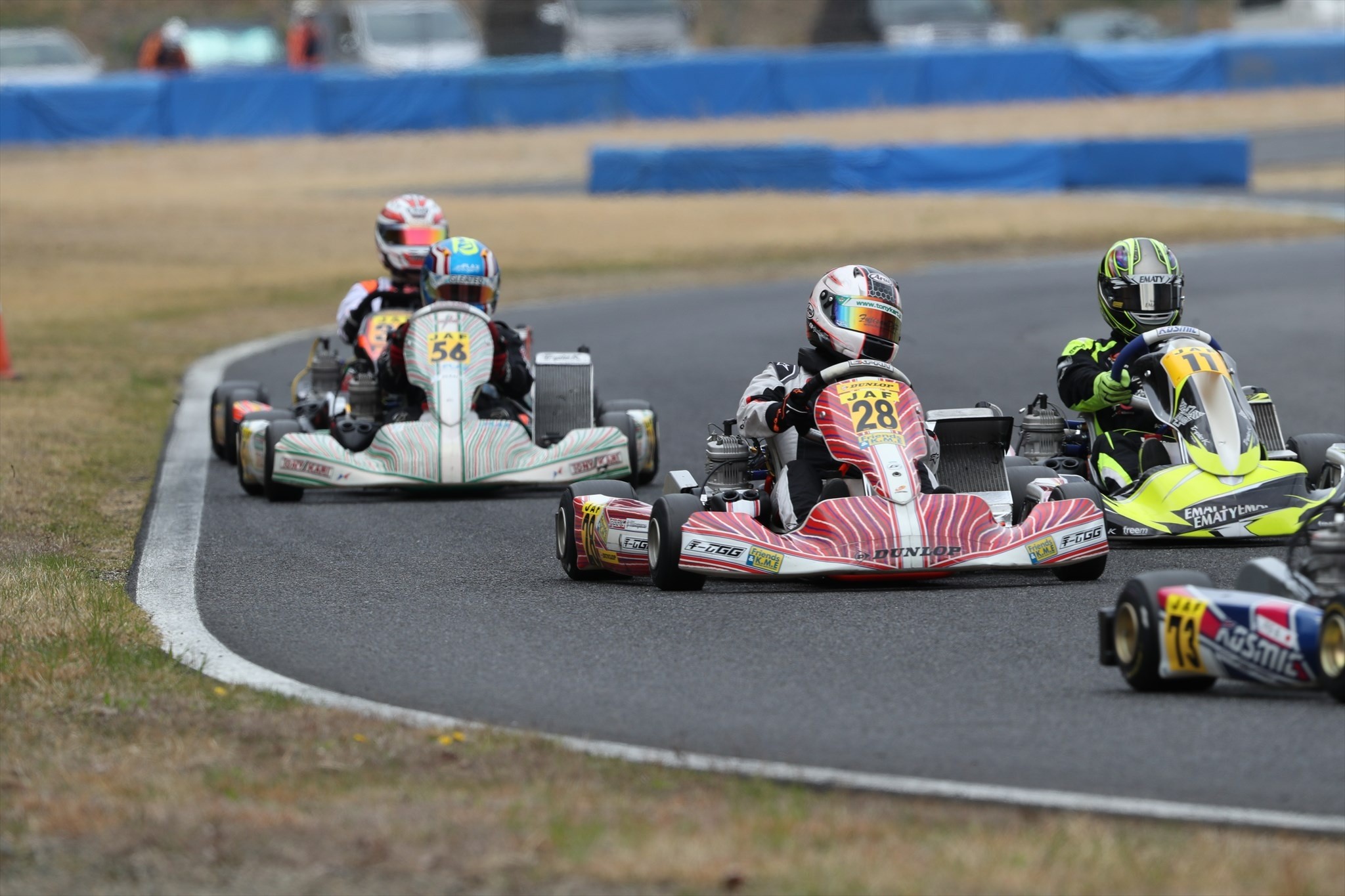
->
[1098,238,1185,341]
[806,264,903,363]
[375,193,449,273]
[420,236,501,314]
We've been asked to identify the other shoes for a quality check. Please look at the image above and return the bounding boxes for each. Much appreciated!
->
[819,478,850,501]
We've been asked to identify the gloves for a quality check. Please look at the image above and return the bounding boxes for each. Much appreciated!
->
[344,309,364,337]
[1097,367,1133,406]
[779,387,811,421]
[390,321,411,367]
[494,337,508,369]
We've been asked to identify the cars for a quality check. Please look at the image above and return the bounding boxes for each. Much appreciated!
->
[813,0,1019,47]
[476,2,697,65]
[283,0,486,76]
[0,26,103,84]
[139,25,289,75]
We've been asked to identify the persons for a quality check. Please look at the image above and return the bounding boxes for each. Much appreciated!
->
[736,264,957,536]
[348,231,536,421]
[282,0,322,68]
[336,195,451,382]
[138,16,190,72]
[1056,237,1267,495]
